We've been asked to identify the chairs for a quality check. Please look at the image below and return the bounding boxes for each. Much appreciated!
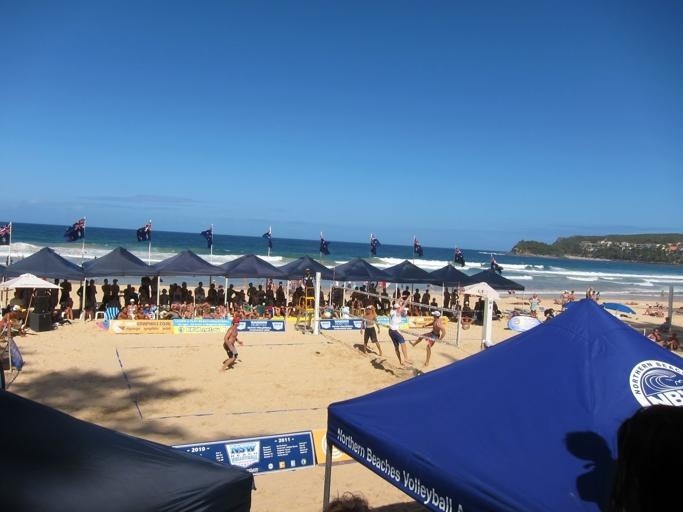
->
[95,302,106,320]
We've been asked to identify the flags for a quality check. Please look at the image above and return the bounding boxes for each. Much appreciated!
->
[137,224,152,242]
[455,247,465,267]
[200,228,213,248]
[0,224,10,245]
[64,218,85,243]
[371,234,381,255]
[490,258,503,274]
[262,233,272,249]
[320,237,329,255]
[414,238,423,256]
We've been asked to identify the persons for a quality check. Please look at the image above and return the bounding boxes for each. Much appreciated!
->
[528,286,600,323]
[408,312,446,367]
[644,301,680,351]
[389,294,413,367]
[360,305,383,356]
[222,316,243,370]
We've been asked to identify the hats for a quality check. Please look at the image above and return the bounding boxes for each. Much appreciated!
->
[130,299,134,301]
[431,311,441,317]
[366,305,372,309]
[393,303,401,309]
